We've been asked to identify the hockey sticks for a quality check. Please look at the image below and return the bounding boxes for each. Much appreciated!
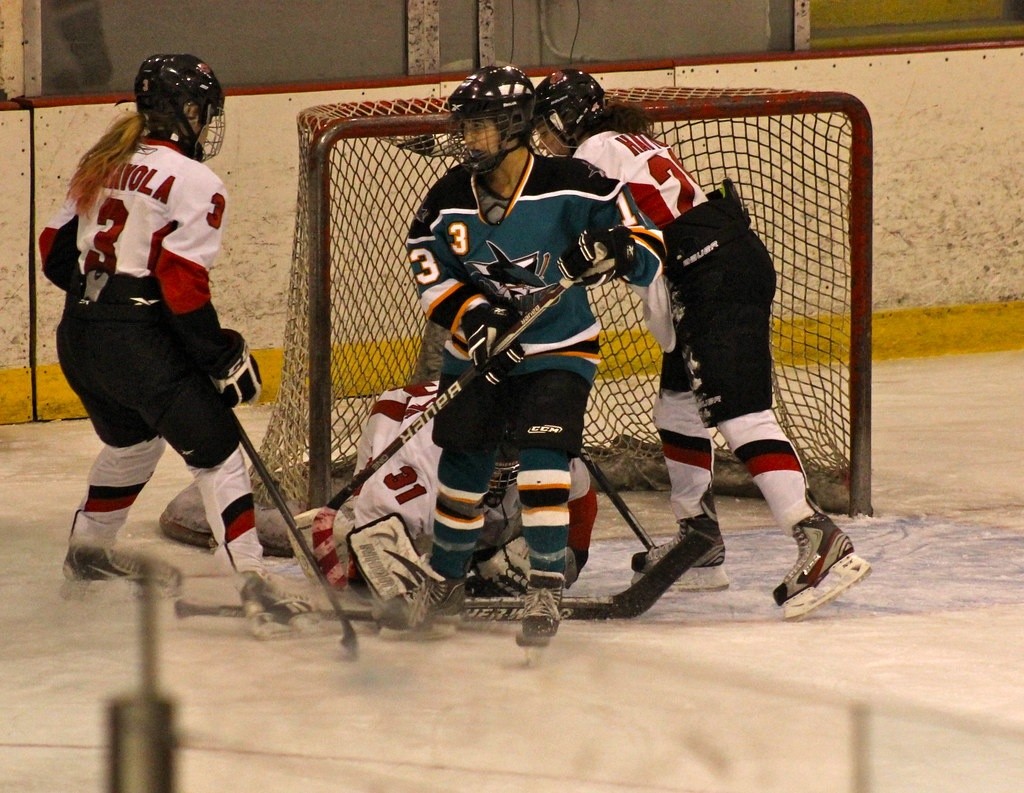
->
[224,402,360,657]
[582,448,657,551]
[174,529,714,622]
[310,239,607,587]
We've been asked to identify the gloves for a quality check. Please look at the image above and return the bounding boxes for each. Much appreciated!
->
[557,224,638,293]
[459,303,525,387]
[205,329,261,407]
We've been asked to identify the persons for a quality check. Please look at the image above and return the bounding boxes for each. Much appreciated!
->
[36,52,326,640]
[320,65,874,668]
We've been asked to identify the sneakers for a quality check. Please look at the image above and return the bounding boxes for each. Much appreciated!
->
[516,569,567,667]
[773,487,872,622]
[630,485,731,593]
[234,570,326,639]
[369,552,468,641]
[59,542,184,602]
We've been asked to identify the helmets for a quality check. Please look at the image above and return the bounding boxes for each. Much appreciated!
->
[448,65,533,176]
[135,54,226,164]
[530,68,605,158]
